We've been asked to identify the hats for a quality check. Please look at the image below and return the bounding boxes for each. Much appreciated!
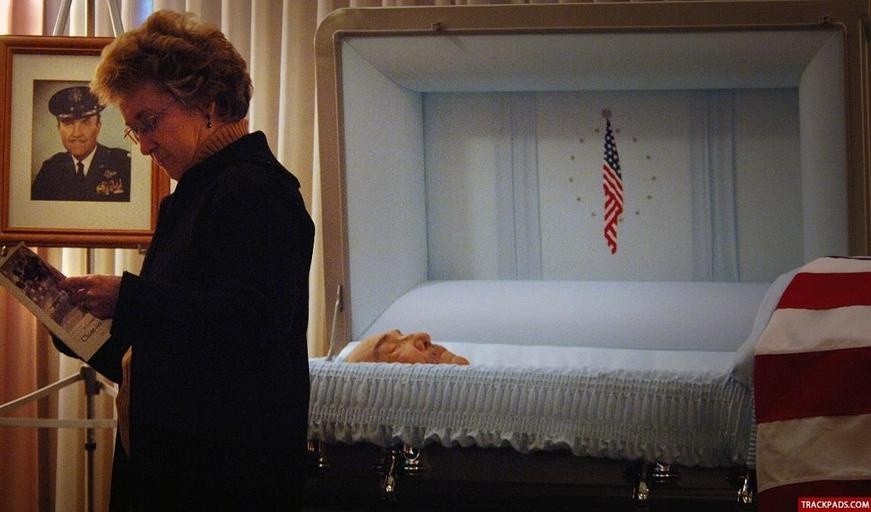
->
[49,86,106,120]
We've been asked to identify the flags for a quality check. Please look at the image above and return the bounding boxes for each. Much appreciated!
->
[603,117,624,257]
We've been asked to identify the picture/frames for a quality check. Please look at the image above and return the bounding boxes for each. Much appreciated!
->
[0,34,170,250]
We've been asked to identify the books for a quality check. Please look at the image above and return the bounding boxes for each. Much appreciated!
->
[1,242,114,362]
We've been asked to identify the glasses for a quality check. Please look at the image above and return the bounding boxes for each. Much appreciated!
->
[123,99,179,146]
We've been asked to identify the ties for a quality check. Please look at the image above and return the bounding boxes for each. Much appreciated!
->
[77,162,84,177]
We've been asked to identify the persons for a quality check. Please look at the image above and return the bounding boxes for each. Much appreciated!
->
[41,9,317,511]
[31,85,130,201]
[341,329,469,367]
